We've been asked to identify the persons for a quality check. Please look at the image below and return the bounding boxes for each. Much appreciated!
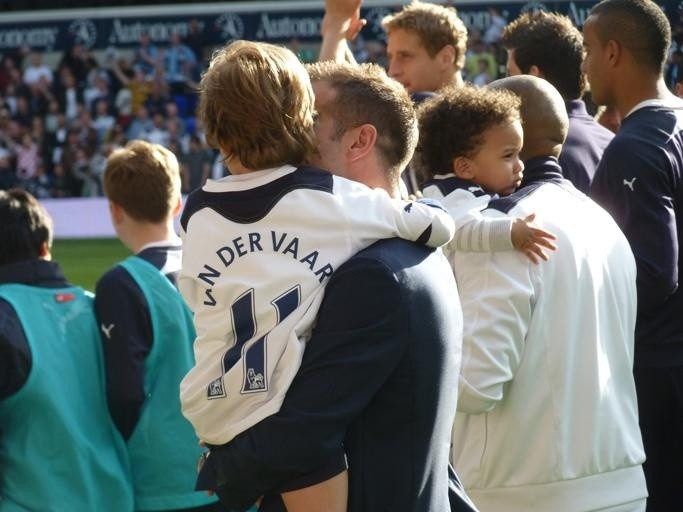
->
[0,0,683,512]
